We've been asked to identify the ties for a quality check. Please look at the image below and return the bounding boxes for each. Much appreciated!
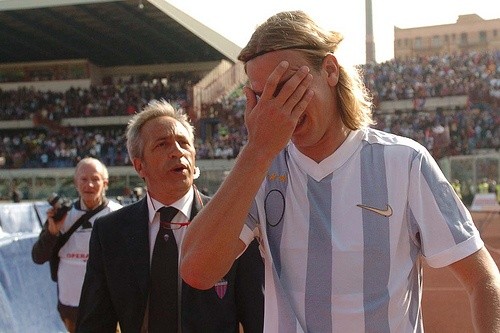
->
[149,207,180,333]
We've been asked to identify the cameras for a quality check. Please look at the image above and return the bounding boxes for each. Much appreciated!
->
[47,192,73,222]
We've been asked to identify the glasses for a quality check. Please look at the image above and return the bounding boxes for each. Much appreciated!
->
[146,183,204,229]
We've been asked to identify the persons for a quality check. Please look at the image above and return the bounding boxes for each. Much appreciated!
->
[74,99,264,333]
[180,8,500,333]
[0,47,499,209]
[31,156,124,333]
